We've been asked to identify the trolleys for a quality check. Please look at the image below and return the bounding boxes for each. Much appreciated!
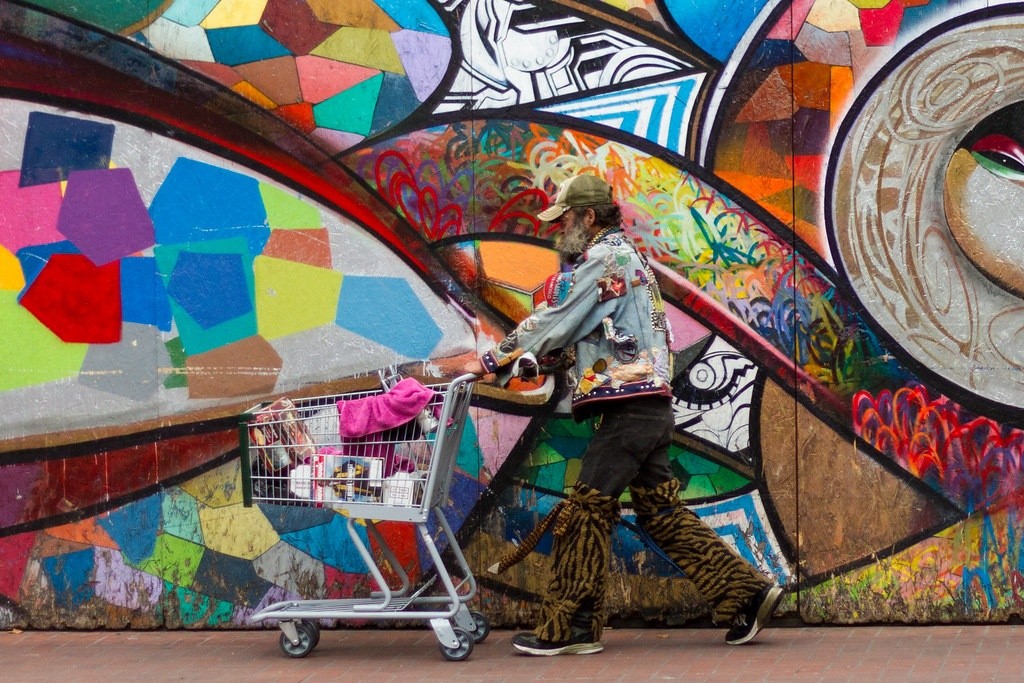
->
[238,372,489,661]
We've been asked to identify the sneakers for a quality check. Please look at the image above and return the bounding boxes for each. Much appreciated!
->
[511,632,604,656]
[725,585,784,644]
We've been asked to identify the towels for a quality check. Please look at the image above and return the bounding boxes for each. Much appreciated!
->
[316,376,454,473]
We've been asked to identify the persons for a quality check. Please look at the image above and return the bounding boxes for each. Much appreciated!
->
[464,174,784,657]
[317,460,381,498]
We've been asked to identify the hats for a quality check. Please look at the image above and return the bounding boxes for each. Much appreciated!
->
[537,173,614,221]
